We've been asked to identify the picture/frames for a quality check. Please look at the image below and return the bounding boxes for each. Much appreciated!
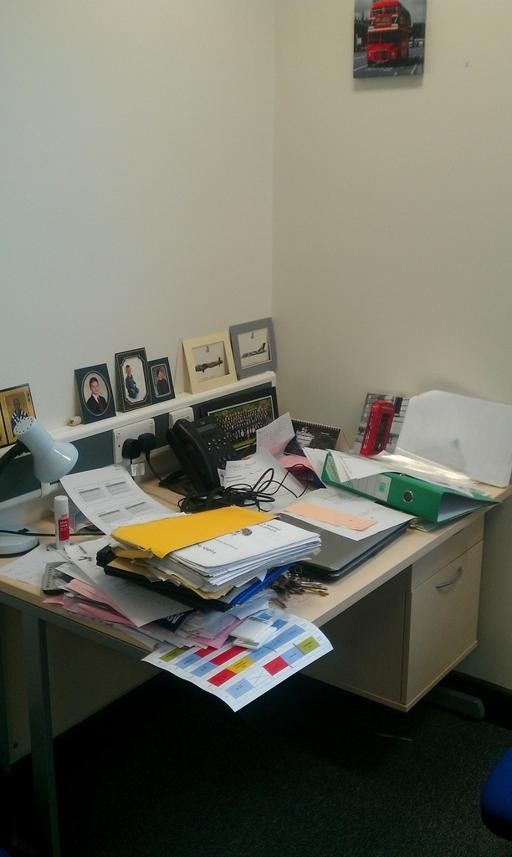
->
[182,331,238,395]
[116,346,152,414]
[74,362,116,426]
[0,384,37,446]
[229,317,278,380]
[147,356,175,404]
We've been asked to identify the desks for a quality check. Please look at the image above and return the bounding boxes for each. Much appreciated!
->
[0,469,511,857]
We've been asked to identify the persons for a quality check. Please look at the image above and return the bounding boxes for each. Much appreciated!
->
[85,377,108,415]
[11,397,28,437]
[216,405,273,445]
[126,364,139,398]
[154,367,170,395]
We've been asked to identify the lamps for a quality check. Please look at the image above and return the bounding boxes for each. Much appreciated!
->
[0,415,79,556]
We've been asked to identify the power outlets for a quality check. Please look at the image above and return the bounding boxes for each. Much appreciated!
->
[113,419,156,464]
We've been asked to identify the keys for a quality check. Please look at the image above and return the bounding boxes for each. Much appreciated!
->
[270,567,329,609]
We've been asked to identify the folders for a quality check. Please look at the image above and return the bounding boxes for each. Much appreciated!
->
[322,453,501,524]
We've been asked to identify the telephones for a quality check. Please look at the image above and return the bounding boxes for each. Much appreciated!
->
[169,416,239,494]
